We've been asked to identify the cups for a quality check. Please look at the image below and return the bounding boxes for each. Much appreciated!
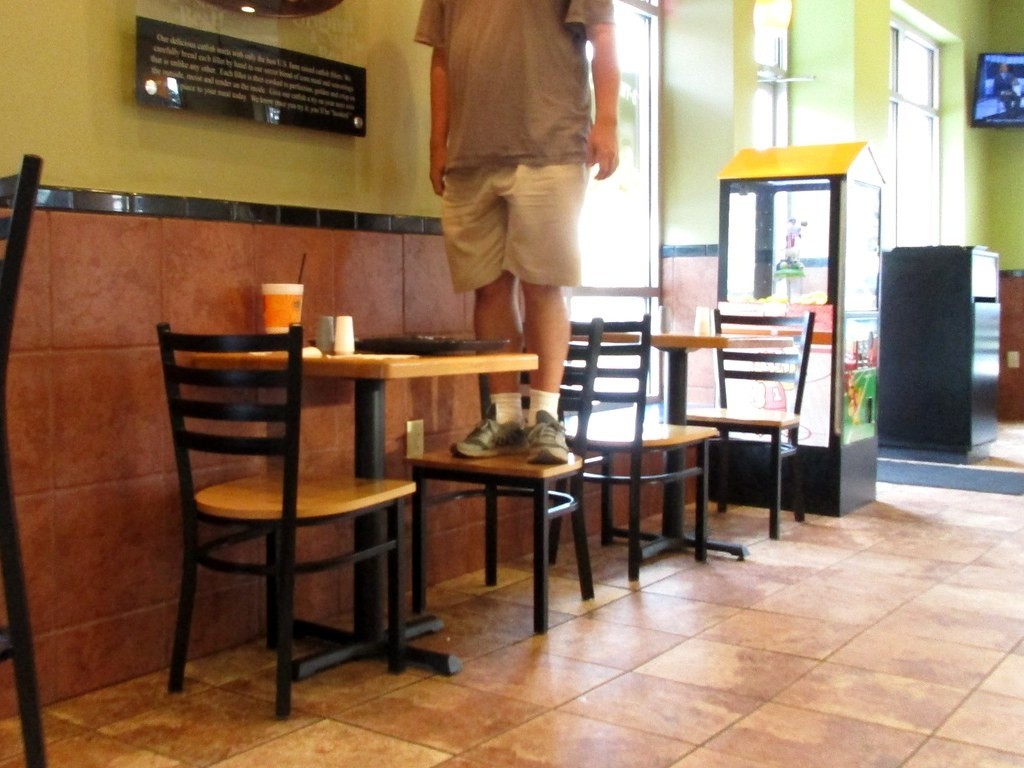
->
[260,283,305,334]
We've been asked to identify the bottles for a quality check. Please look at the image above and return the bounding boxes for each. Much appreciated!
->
[694,306,710,336]
[658,306,672,334]
[333,315,356,355]
[316,315,334,353]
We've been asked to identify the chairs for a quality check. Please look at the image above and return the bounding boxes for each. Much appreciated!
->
[155,317,415,721]
[685,309,815,540]
[400,316,604,634]
[550,314,718,582]
[0,156,76,768]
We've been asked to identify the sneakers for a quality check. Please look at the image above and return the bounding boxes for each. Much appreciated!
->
[523,409,570,464]
[450,403,529,457]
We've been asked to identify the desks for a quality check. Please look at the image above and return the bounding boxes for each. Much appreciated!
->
[570,330,794,565]
[194,351,540,681]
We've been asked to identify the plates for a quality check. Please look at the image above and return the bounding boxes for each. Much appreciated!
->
[357,337,511,356]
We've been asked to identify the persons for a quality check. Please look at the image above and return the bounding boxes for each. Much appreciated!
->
[415,0,619,463]
[994,65,1021,116]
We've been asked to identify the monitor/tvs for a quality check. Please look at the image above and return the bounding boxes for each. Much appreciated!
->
[970,53,1024,129]
